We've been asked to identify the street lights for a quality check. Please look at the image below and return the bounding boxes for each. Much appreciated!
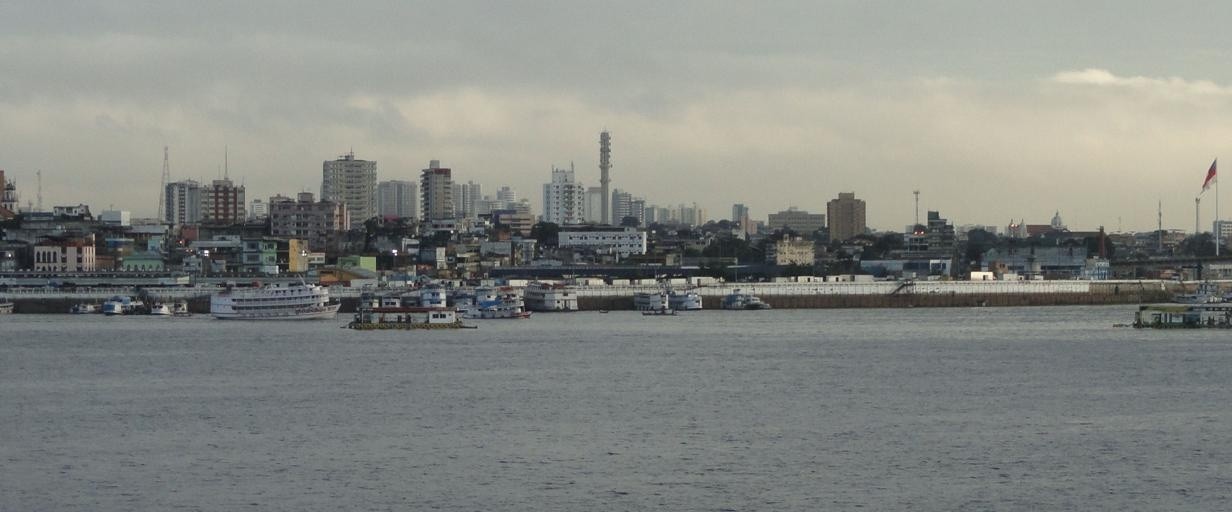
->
[301,251,307,274]
[203,250,209,274]
[391,249,398,267]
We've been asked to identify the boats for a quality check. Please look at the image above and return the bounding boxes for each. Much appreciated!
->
[524,279,580,311]
[635,292,703,316]
[1134,281,1232,329]
[720,294,771,311]
[0,301,16,314]
[462,285,534,320]
[350,280,461,328]
[210,280,342,320]
[71,296,188,316]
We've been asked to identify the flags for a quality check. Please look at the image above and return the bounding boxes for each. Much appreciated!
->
[1202,159,1216,188]
